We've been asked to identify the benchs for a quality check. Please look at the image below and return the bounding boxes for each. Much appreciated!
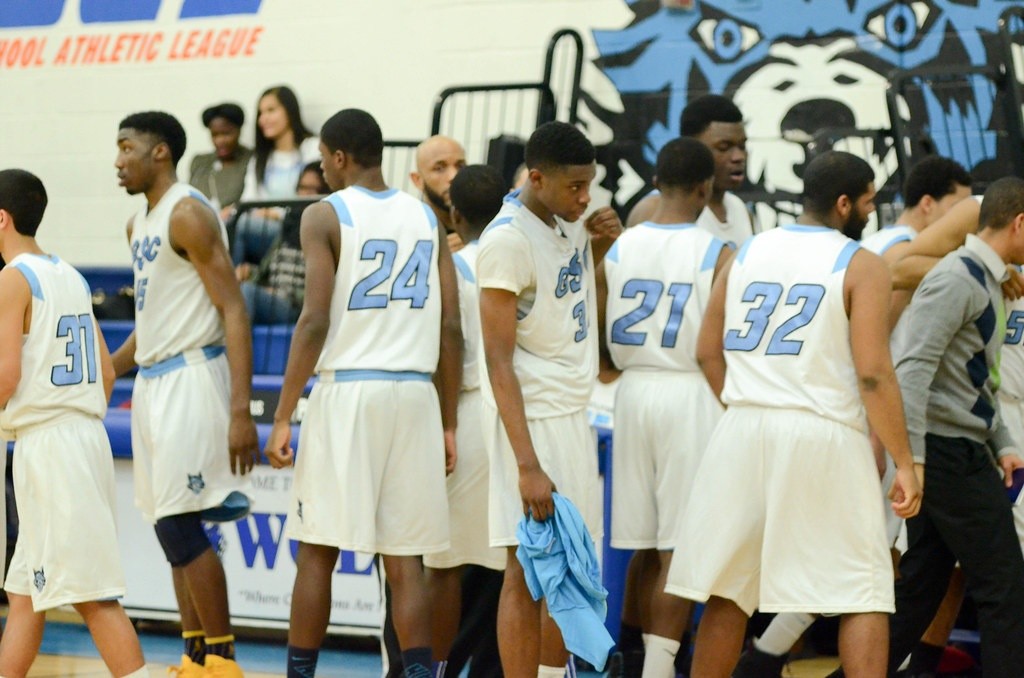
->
[94,316,317,393]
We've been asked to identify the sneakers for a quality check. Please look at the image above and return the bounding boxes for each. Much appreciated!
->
[169,653,246,678]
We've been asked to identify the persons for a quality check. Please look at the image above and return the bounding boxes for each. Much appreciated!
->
[377,122,623,678]
[109,111,260,677]
[665,151,924,678]
[729,155,1024,677]
[190,85,333,326]
[595,94,748,678]
[264,108,465,678]
[0,169,150,678]
